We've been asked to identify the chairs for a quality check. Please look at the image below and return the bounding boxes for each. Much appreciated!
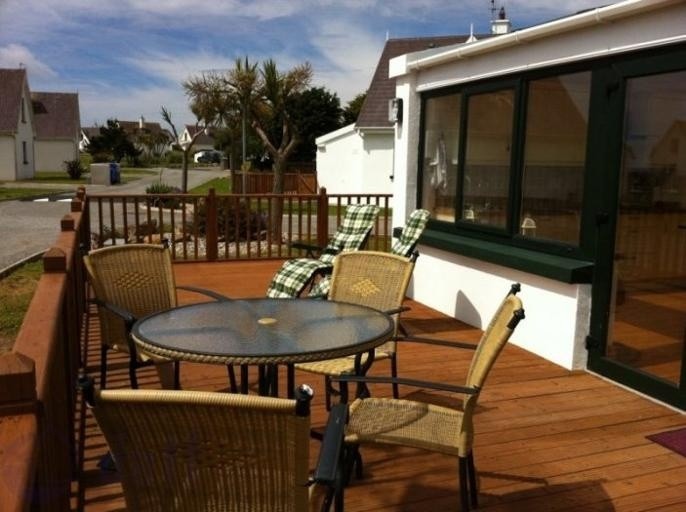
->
[309,209,432,336]
[328,281,526,511]
[308,203,381,296]
[288,245,420,402]
[84,238,182,391]
[265,258,333,298]
[85,379,315,512]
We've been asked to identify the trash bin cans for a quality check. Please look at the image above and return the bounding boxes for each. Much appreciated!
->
[221,157,228,170]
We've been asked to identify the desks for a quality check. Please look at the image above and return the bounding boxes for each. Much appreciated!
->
[130,296,395,398]
